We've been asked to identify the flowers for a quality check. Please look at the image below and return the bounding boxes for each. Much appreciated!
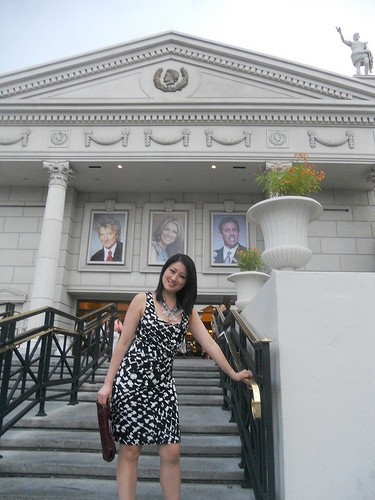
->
[255,152,326,196]
[236,248,261,271]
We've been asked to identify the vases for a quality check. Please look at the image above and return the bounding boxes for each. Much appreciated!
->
[227,272,271,310]
[247,196,324,271]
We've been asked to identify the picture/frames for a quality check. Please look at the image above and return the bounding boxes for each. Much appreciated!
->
[210,213,249,267]
[86,211,128,265]
[147,211,187,266]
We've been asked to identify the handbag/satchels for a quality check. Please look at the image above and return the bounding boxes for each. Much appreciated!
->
[96,397,116,463]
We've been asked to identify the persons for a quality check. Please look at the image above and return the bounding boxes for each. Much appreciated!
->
[336,26,370,76]
[212,216,247,264]
[100,317,124,356]
[151,216,185,265]
[90,216,123,262]
[96,253,254,500]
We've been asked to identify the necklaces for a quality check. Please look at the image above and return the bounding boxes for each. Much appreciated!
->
[160,302,179,321]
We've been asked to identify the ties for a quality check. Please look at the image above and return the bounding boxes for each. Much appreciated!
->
[106,251,113,261]
[224,251,232,263]
